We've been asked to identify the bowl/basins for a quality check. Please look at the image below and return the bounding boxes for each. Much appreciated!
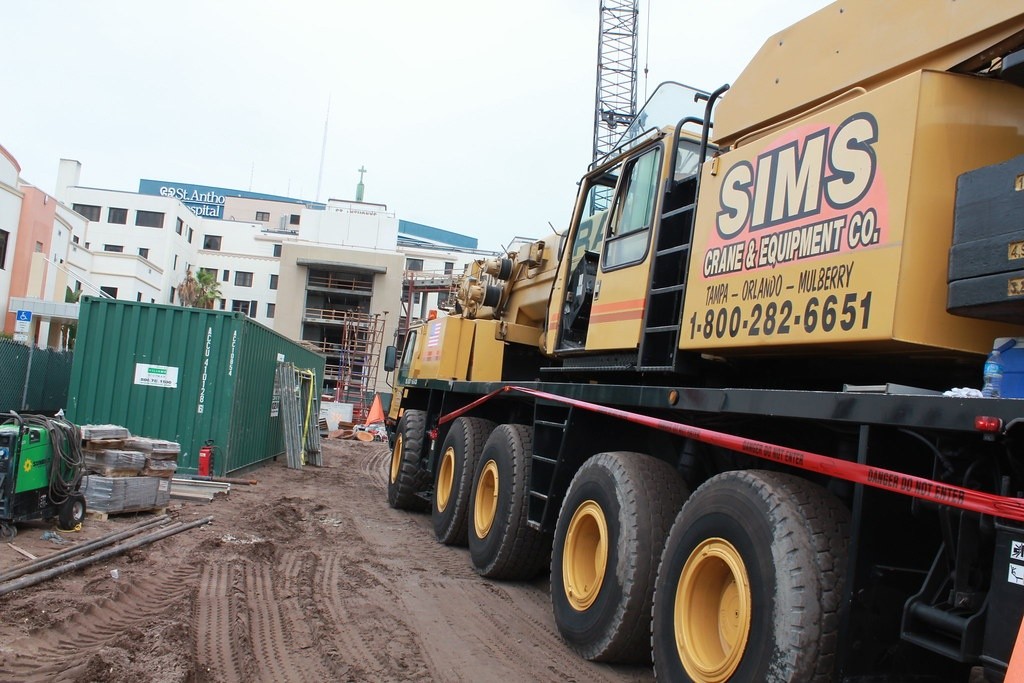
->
[338,430,354,439]
[356,431,374,441]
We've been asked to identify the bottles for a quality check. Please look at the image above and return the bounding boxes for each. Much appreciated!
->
[982,351,1003,397]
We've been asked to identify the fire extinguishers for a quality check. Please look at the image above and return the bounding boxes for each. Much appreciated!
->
[198,439,225,478]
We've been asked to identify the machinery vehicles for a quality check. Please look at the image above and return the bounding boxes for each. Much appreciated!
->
[381,1,1024,681]
